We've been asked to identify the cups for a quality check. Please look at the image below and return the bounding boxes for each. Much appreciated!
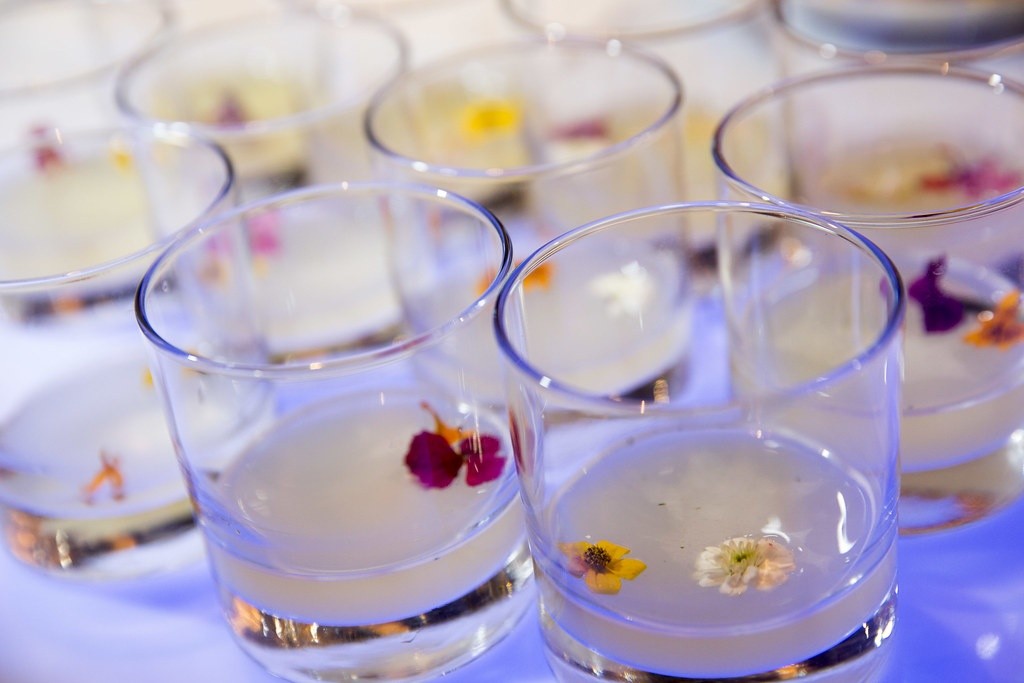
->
[109,13,436,383]
[0,1,188,329]
[760,1,1022,294]
[362,36,694,480]
[133,183,514,683]
[0,123,276,579]
[710,61,1024,537]
[497,0,791,292]
[493,200,904,682]
